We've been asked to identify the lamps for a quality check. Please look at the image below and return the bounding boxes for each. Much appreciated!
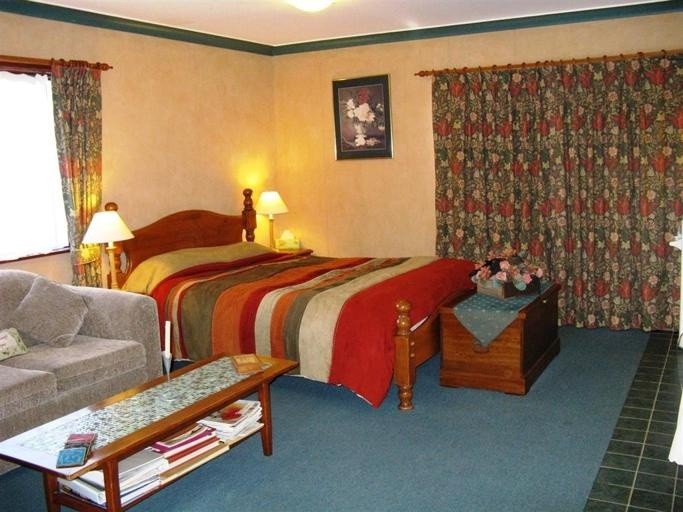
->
[81,210,136,290]
[253,192,289,248]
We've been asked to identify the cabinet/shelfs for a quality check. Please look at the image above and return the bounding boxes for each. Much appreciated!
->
[438,283,562,396]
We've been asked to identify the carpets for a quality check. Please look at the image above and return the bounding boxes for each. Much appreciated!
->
[0,324,649,512]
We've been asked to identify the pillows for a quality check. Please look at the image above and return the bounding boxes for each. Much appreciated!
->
[7,276,94,348]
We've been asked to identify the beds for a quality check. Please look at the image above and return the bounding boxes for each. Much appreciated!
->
[104,188,480,411]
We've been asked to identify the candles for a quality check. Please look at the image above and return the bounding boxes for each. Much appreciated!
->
[165,320,171,359]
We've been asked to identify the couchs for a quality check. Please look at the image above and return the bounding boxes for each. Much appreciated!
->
[0,269,163,476]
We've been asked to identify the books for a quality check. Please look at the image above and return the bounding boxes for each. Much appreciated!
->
[55,398,267,511]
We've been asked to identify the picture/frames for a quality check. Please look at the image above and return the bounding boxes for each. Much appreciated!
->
[330,72,394,162]
